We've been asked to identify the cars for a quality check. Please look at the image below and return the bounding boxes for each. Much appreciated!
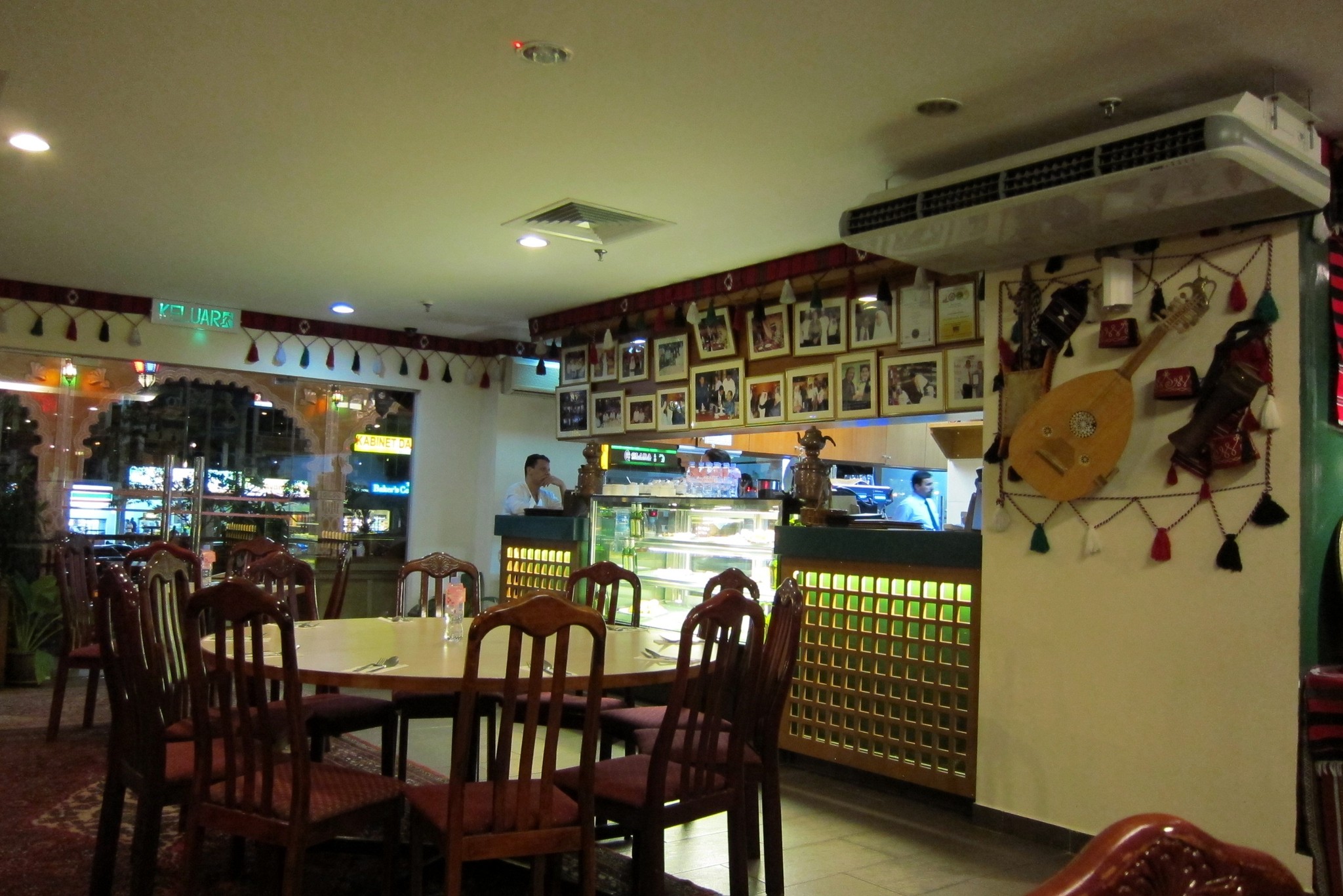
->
[84,544,147,581]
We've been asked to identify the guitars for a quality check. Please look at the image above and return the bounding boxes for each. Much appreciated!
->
[1006,276,1219,503]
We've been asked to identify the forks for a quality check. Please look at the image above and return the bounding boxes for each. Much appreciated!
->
[294,623,319,628]
[659,636,699,642]
[526,661,554,675]
[641,652,677,661]
[401,617,412,621]
[210,633,267,639]
[352,657,388,672]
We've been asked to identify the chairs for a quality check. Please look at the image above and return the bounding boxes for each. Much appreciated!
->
[37,522,806,896]
[1025,810,1306,896]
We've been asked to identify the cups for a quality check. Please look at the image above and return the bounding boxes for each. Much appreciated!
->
[602,479,681,497]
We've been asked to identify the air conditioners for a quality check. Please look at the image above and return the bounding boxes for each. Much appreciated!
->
[502,355,561,399]
[839,87,1333,277]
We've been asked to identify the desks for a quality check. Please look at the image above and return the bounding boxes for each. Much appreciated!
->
[202,610,719,896]
[96,579,305,719]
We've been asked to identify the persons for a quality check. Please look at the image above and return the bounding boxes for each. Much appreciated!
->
[561,309,983,432]
[501,454,567,515]
[891,470,944,531]
[700,448,732,467]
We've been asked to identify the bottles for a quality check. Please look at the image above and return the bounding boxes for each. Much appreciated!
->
[763,604,771,645]
[702,462,713,498]
[769,548,777,590]
[721,463,730,497]
[685,462,696,497]
[696,462,706,497]
[443,577,464,642]
[622,502,644,574]
[730,463,742,497]
[594,582,609,620]
[200,544,212,587]
[713,462,721,498]
[356,541,364,557]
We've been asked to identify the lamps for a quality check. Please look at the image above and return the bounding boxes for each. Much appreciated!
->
[133,361,160,392]
[60,360,78,389]
[330,386,344,411]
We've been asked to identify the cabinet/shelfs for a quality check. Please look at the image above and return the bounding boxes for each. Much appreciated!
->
[585,490,862,646]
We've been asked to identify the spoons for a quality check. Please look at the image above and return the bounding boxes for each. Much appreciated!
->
[245,644,300,655]
[295,621,312,628]
[543,660,572,675]
[366,656,399,672]
[383,616,402,622]
[645,648,678,660]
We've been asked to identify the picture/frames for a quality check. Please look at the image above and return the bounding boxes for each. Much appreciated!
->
[617,336,649,385]
[847,287,898,351]
[745,302,792,362]
[590,338,619,383]
[688,356,746,431]
[935,279,978,345]
[652,332,690,384]
[625,392,656,432]
[879,349,946,417]
[834,349,878,421]
[655,385,691,433]
[975,270,985,339]
[556,381,591,440]
[792,295,849,357]
[692,306,738,360]
[559,343,590,388]
[785,362,835,424]
[896,281,935,352]
[744,372,786,427]
[943,343,984,412]
[590,387,626,436]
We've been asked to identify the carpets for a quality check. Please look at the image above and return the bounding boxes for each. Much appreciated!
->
[0,720,722,896]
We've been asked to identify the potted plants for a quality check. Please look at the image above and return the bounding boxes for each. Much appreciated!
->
[0,562,72,686]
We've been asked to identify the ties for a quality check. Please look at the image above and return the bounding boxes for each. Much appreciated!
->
[925,501,939,530]
